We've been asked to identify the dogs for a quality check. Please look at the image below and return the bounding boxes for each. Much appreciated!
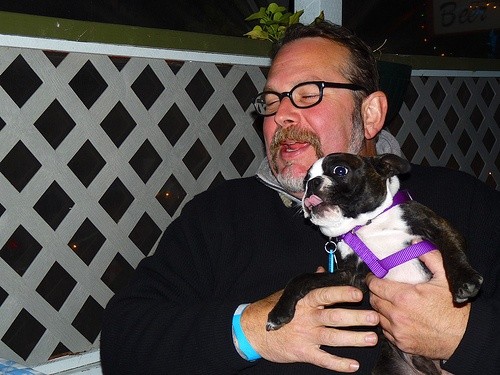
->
[264,151,500,372]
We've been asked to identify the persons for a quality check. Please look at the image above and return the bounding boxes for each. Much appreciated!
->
[101,24,500,375]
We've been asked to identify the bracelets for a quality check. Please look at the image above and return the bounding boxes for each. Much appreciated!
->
[233,303,261,361]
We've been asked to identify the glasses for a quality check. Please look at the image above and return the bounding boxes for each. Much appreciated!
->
[252,81,369,117]
